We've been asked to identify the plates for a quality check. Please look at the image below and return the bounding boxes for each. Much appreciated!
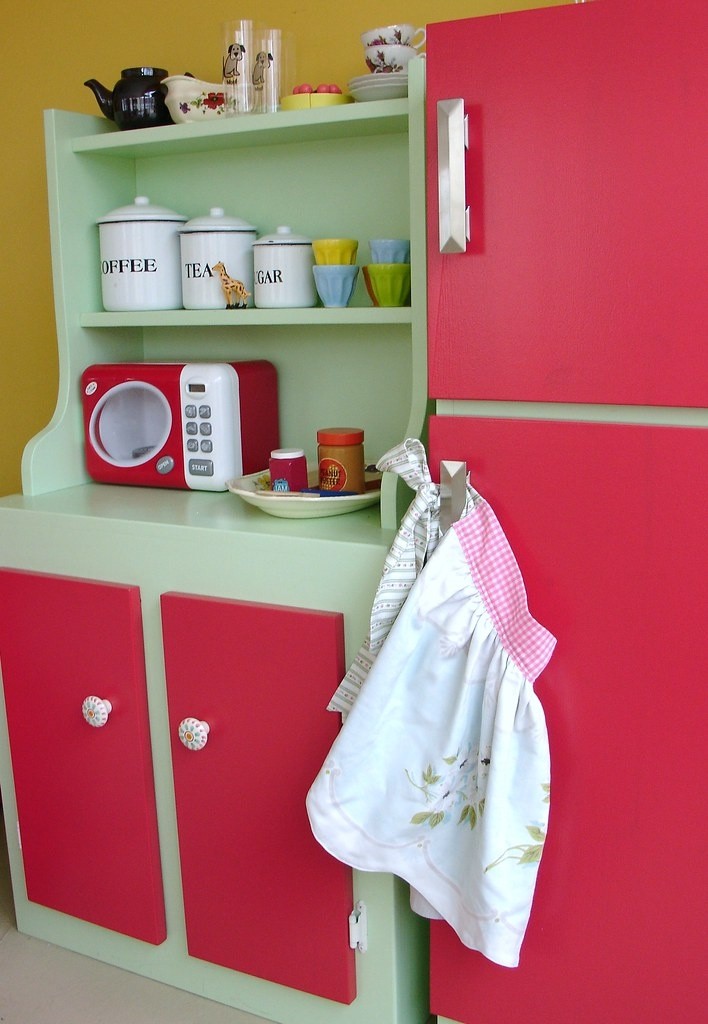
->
[226,462,380,518]
[347,70,408,102]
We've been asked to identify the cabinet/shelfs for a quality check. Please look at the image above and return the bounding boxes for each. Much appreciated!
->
[0,51,428,1024]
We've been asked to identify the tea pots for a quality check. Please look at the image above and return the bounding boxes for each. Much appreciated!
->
[83,66,196,130]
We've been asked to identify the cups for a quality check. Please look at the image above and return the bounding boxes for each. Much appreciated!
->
[364,45,417,73]
[368,238,410,265]
[362,23,427,48]
[95,195,190,311]
[312,238,359,264]
[312,264,360,309]
[369,264,411,307]
[178,207,260,310]
[252,225,317,308]
[362,266,380,308]
[252,29,282,113]
[221,20,253,118]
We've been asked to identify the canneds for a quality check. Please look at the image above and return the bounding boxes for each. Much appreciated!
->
[269,448,308,492]
[316,427,365,494]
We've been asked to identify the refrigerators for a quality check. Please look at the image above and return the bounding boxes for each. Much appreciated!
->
[426,0,708,1024]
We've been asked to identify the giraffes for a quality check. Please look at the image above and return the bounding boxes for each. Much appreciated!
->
[212,261,252,309]
